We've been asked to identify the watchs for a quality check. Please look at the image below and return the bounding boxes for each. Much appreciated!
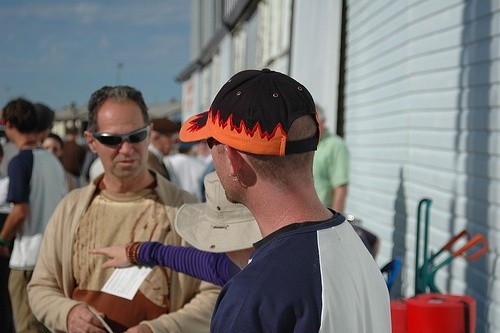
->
[0,236,8,246]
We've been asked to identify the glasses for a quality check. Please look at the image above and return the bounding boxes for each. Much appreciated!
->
[88,123,152,145]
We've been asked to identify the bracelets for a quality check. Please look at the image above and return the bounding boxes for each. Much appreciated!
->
[126,241,146,265]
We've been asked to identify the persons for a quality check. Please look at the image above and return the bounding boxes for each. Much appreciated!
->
[89,170,264,287]
[1,98,104,333]
[147,70,392,333]
[27,84,223,332]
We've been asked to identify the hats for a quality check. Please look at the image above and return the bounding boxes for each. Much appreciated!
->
[179,66,321,154]
[176,172,265,253]
